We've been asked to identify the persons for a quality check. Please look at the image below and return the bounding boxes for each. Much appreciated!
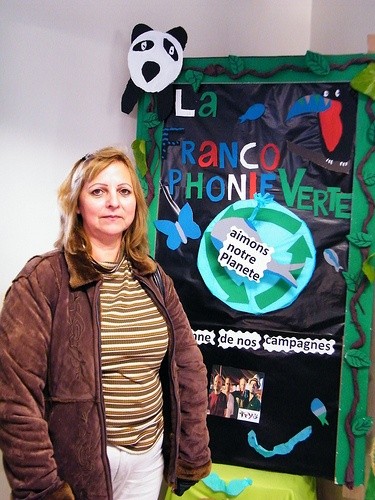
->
[0,144,211,500]
[208,371,262,421]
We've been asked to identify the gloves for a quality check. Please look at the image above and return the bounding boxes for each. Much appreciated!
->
[173,478,198,496]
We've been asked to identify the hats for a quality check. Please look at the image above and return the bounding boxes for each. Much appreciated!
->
[249,374,261,386]
